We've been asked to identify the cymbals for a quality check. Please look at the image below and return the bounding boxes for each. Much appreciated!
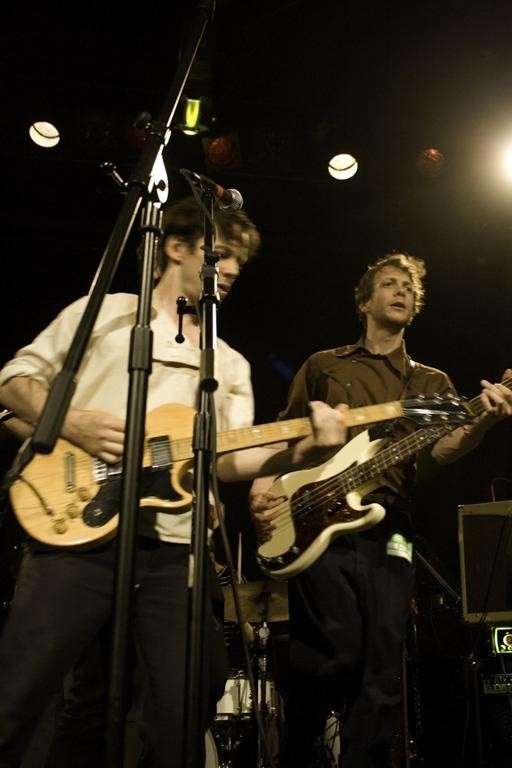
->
[221,578,290,625]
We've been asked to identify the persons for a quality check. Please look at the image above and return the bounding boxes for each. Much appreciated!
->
[239,252,512,768]
[207,538,250,650]
[0,186,352,768]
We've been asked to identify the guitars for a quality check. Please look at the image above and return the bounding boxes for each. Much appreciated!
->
[10,393,473,553]
[250,379,511,582]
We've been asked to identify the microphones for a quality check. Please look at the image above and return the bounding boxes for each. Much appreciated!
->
[190,170,245,213]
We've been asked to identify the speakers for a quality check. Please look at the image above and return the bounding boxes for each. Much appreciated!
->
[456,500,511,625]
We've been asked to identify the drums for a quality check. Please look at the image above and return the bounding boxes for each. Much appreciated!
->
[206,670,272,716]
[204,725,263,768]
